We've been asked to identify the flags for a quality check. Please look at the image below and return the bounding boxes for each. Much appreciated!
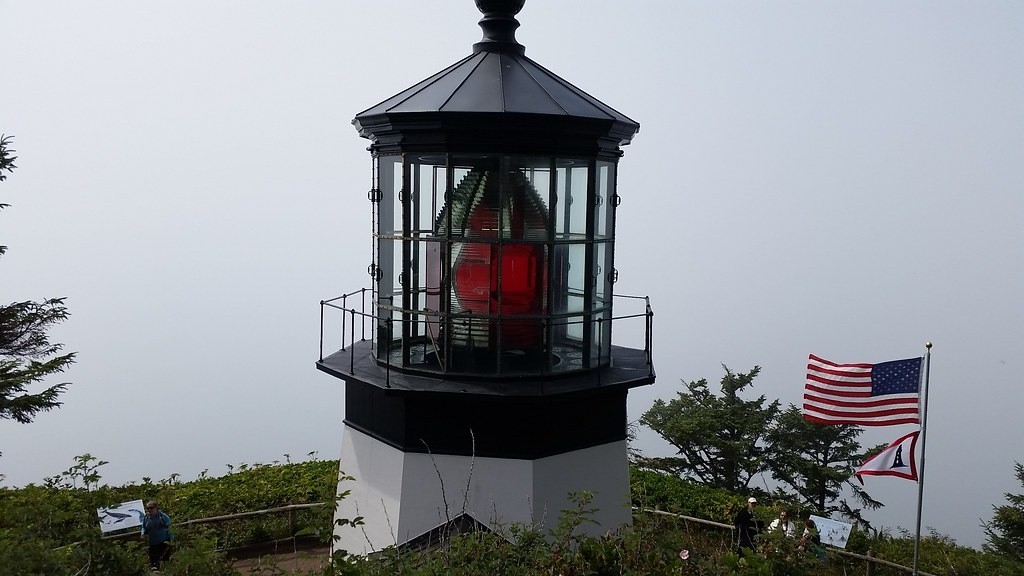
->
[803,353,926,427]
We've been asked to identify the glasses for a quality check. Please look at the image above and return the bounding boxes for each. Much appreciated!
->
[146,506,155,510]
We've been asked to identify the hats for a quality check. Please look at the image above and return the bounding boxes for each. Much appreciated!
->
[748,498,757,504]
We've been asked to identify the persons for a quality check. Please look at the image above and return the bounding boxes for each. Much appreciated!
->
[734,498,820,559]
[140,501,175,571]
